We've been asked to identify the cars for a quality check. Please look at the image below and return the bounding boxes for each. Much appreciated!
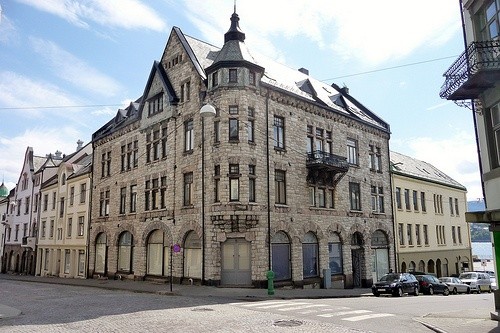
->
[439,277,470,294]
[414,275,449,295]
[489,275,497,292]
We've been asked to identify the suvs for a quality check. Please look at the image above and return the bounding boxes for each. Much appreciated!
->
[371,271,419,297]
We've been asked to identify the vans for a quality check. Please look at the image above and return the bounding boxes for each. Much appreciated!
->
[459,271,492,294]
